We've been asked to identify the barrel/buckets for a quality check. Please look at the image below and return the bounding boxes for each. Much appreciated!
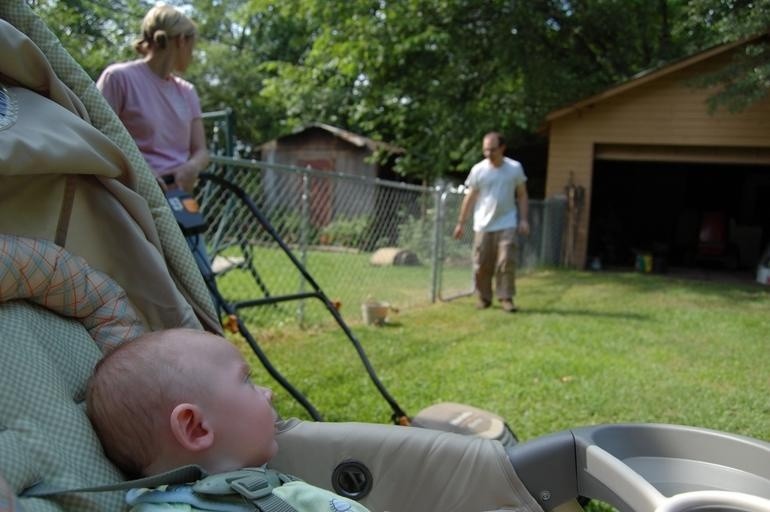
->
[757,254,770,283]
[361,296,391,324]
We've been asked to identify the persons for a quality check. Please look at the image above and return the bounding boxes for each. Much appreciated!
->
[84,330,381,512]
[452,132,530,312]
[94,2,219,322]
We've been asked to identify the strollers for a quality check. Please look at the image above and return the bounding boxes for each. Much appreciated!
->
[1,0,770,511]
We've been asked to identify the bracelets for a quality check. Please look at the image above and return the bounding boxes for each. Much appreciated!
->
[457,219,467,225]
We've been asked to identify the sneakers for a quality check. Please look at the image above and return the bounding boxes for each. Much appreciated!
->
[476,298,491,310]
[500,297,516,312]
